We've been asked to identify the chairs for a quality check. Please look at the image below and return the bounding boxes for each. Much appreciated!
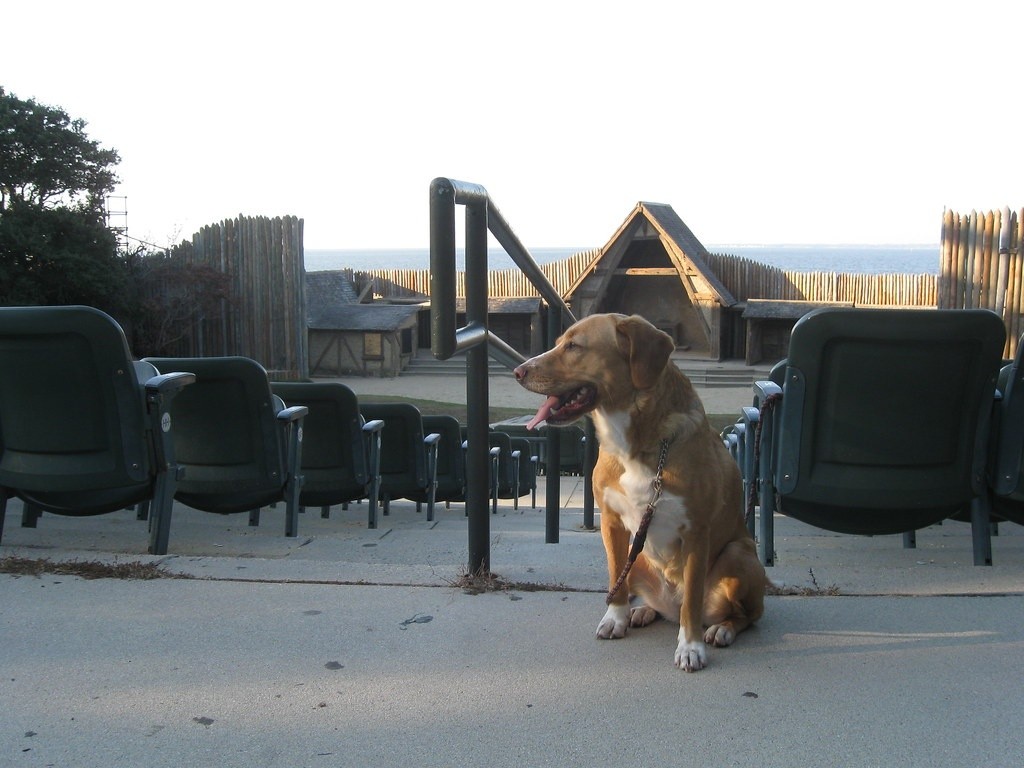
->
[717,307,1024,567]
[0,305,587,555]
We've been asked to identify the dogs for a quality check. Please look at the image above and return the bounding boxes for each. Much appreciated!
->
[514,313,771,674]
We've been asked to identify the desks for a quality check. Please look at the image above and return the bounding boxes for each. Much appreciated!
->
[651,321,680,345]
